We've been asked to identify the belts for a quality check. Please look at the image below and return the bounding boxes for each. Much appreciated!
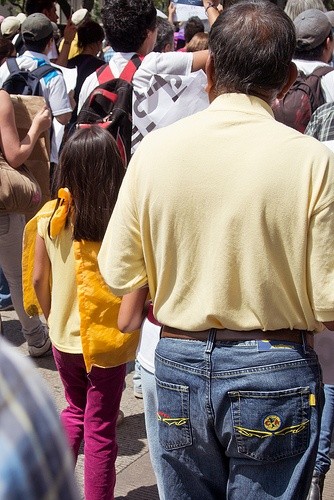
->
[159,326,315,347]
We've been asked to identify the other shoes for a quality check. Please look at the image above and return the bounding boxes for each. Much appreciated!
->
[116,411,124,428]
[28,337,52,358]
[134,390,143,399]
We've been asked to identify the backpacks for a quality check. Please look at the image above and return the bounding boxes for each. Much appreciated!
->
[1,58,63,96]
[58,53,145,168]
[271,66,334,134]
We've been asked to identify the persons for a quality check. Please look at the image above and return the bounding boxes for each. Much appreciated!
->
[97,0,334,500]
[0,0,334,500]
[22,126,145,500]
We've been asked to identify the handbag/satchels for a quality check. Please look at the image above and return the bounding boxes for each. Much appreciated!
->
[0,153,42,213]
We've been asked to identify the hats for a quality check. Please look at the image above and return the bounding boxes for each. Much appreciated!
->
[1,16,20,38]
[71,8,91,29]
[292,8,334,50]
[21,12,58,42]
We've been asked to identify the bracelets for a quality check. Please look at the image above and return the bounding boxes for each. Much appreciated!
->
[205,2,215,17]
